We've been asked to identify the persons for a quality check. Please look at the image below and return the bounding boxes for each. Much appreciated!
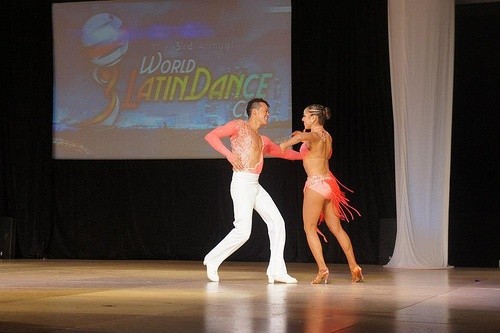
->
[280,103,364,285]
[203,97,310,285]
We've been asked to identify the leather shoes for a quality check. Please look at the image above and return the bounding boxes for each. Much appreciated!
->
[267,275,298,284]
[202,257,219,282]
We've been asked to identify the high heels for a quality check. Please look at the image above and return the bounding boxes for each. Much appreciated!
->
[351,266,365,282]
[311,267,329,284]
